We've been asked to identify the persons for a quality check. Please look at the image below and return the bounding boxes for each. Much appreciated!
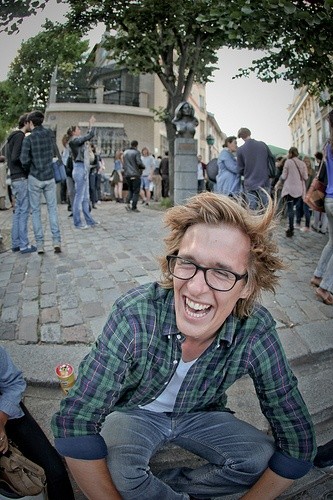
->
[170,101,199,137]
[311,110,333,305]
[0,116,328,237]
[20,110,62,254]
[49,192,319,500]
[0,345,74,500]
[6,112,38,254]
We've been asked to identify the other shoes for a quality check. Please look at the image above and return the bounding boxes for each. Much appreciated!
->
[1,196,327,253]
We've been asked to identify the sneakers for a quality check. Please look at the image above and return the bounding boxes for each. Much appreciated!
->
[310,276,323,288]
[315,286,333,306]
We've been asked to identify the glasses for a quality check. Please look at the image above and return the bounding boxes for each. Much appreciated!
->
[166,248,248,292]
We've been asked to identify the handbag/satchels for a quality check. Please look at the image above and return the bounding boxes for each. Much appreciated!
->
[268,155,276,178]
[53,158,66,184]
